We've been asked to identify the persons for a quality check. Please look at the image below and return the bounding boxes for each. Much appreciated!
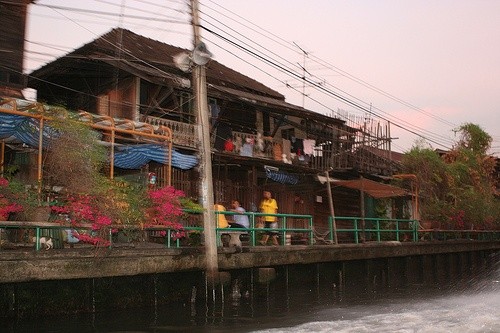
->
[227,200,250,233]
[258,189,280,246]
[214,199,229,228]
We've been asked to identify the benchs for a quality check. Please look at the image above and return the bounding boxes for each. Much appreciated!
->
[218,230,247,247]
